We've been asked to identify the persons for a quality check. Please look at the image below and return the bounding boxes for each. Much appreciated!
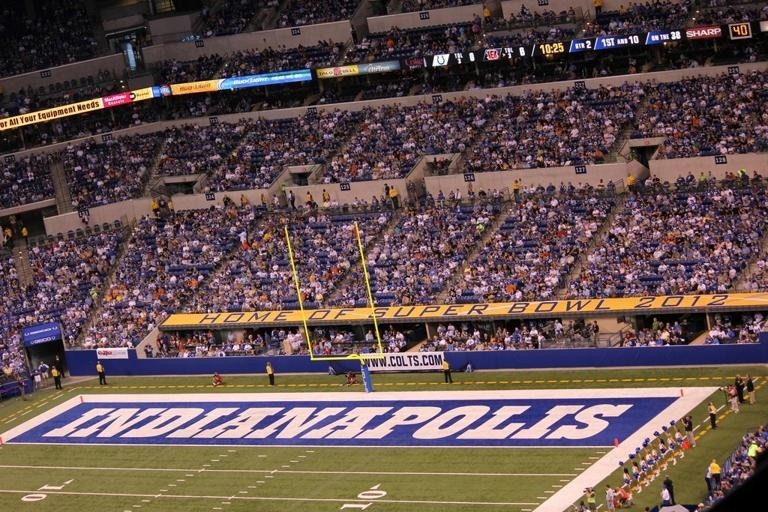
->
[572,391,767,511]
[0,1,768,390]
[18,378,29,403]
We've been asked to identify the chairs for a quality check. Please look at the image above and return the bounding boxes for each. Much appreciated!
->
[0,1,767,359]
